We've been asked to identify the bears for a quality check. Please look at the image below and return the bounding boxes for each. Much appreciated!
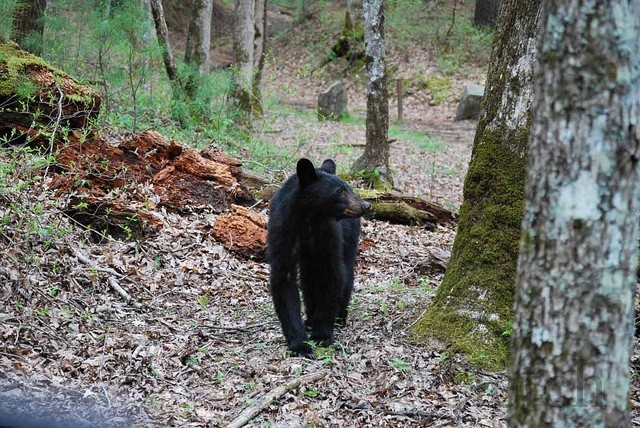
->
[265,158,371,360]
